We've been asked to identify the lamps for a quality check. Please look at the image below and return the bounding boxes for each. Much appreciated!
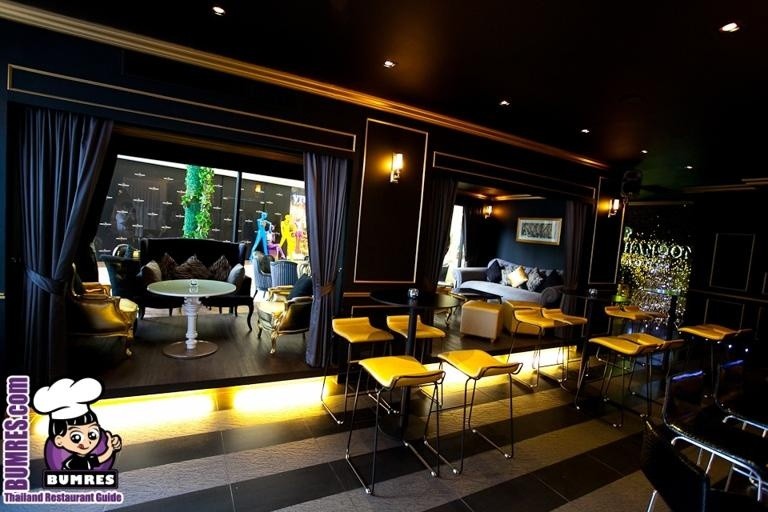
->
[390,151,405,184]
[607,198,620,218]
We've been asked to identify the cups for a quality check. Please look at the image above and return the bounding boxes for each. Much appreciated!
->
[408,288,419,299]
[588,288,598,297]
[190,279,198,287]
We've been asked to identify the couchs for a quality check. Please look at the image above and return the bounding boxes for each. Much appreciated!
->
[453,257,566,307]
[99,236,255,333]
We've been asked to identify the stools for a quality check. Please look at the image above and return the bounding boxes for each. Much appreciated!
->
[318,299,753,495]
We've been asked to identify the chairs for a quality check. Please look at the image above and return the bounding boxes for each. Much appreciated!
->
[635,357,768,511]
[255,285,314,355]
[67,260,140,357]
[268,260,298,287]
[436,282,465,325]
[253,254,275,299]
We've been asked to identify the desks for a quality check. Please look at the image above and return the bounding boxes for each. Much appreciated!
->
[452,287,503,305]
[147,278,238,360]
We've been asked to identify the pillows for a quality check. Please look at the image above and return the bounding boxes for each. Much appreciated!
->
[72,274,87,294]
[542,269,563,291]
[142,258,161,283]
[484,259,503,283]
[206,255,230,280]
[175,254,209,280]
[525,266,545,291]
[226,262,245,295]
[505,266,527,288]
[161,252,178,280]
[286,272,314,303]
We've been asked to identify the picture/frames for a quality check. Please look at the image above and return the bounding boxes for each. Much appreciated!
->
[514,217,564,247]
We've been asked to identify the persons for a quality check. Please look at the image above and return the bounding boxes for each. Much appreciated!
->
[249,213,302,260]
[115,201,137,232]
